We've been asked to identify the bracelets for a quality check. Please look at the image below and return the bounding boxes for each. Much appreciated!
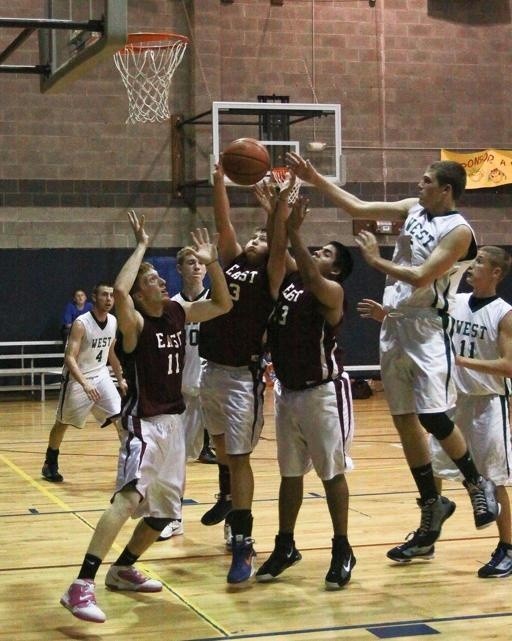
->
[205,258,219,266]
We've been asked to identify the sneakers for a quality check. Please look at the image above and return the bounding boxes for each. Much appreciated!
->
[478,544,512,579]
[387,531,436,563]
[416,494,455,548]
[255,536,302,583]
[60,578,107,624]
[160,513,186,539]
[324,537,358,591]
[462,475,500,529]
[225,535,257,584]
[198,452,217,461]
[222,523,234,553]
[200,493,234,526]
[105,565,163,593]
[41,459,64,482]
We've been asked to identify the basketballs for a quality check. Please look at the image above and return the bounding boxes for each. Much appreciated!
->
[221,137,270,186]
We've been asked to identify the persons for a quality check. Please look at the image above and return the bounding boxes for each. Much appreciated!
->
[355,245,512,579]
[60,286,93,345]
[253,171,357,590]
[284,150,499,546]
[60,209,235,624]
[198,157,296,587]
[41,281,127,482]
[150,245,219,541]
